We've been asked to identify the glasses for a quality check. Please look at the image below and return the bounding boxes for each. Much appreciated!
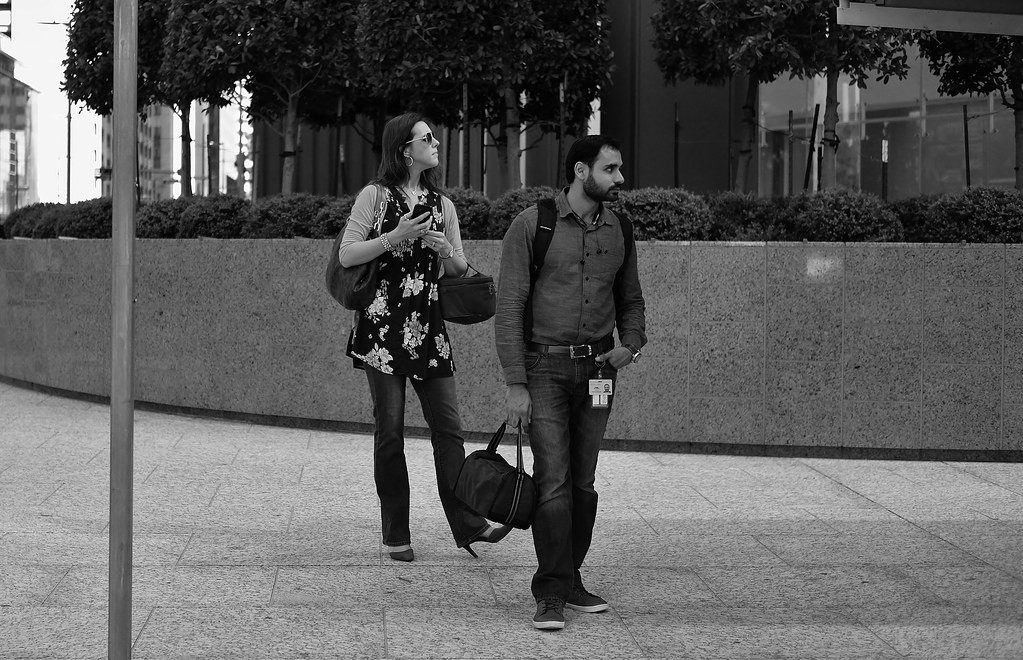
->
[406,131,435,144]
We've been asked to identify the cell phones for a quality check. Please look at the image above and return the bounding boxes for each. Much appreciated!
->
[411,204,433,224]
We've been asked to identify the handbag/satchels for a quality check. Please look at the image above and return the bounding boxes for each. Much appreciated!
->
[438,262,496,325]
[454,418,536,530]
[326,184,384,310]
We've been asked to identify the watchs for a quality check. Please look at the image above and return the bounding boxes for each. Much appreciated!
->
[625,344,642,364]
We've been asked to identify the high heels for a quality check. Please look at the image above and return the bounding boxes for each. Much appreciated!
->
[389,549,414,561]
[463,525,513,558]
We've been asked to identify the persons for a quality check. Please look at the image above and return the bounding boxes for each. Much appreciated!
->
[494,133,651,634]
[603,384,611,393]
[337,113,514,564]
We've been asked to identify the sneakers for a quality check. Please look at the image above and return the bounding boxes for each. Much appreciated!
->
[565,585,608,612]
[532,599,565,629]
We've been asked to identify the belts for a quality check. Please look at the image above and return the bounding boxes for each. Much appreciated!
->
[528,336,612,359]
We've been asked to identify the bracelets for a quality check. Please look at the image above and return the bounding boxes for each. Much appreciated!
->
[439,243,454,260]
[380,232,396,253]
